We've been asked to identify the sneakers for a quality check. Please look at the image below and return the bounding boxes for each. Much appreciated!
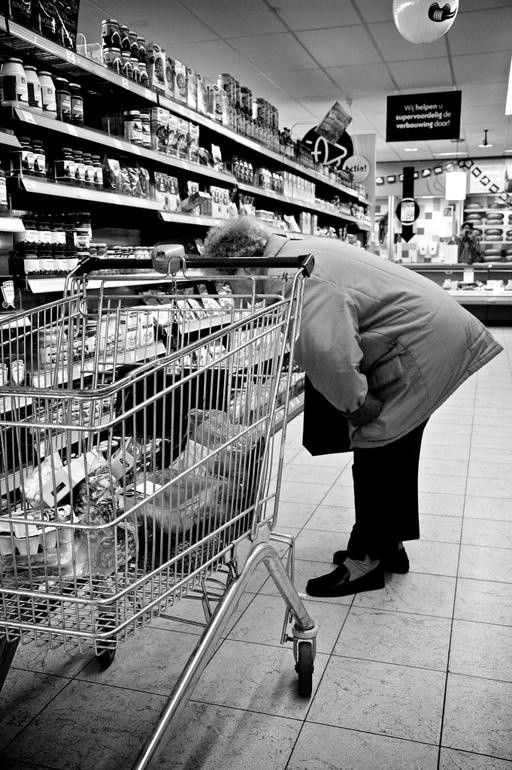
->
[306,561,385,597]
[333,547,410,575]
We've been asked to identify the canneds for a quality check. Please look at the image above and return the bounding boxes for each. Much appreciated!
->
[0,19,302,439]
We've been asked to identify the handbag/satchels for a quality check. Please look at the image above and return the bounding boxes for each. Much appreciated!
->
[301,373,352,456]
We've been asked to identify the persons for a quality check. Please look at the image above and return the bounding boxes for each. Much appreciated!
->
[202,216,505,598]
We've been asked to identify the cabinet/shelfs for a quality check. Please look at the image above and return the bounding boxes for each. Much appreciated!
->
[0,16,369,497]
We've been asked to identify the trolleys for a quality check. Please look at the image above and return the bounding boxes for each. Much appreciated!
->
[0,244,315,765]
